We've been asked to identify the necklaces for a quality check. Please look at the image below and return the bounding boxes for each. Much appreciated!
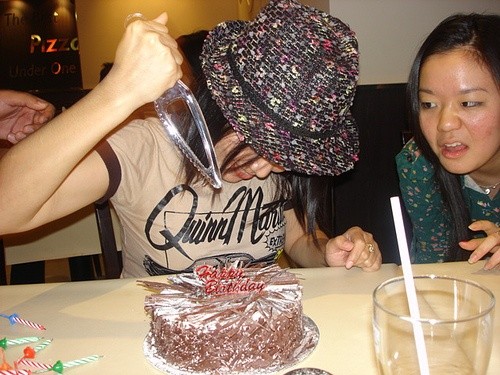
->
[477,181,500,195]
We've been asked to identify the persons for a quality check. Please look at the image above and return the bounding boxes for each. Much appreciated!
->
[0,0,384,280]
[393,12,500,272]
[0,89,56,145]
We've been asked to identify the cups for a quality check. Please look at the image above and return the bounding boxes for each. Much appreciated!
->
[371,273,497,375]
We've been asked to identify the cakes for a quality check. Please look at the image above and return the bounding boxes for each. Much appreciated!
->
[136,261,316,375]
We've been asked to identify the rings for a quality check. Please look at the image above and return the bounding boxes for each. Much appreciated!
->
[367,243,374,253]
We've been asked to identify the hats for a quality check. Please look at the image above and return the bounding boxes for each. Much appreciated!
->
[198,0,361,177]
